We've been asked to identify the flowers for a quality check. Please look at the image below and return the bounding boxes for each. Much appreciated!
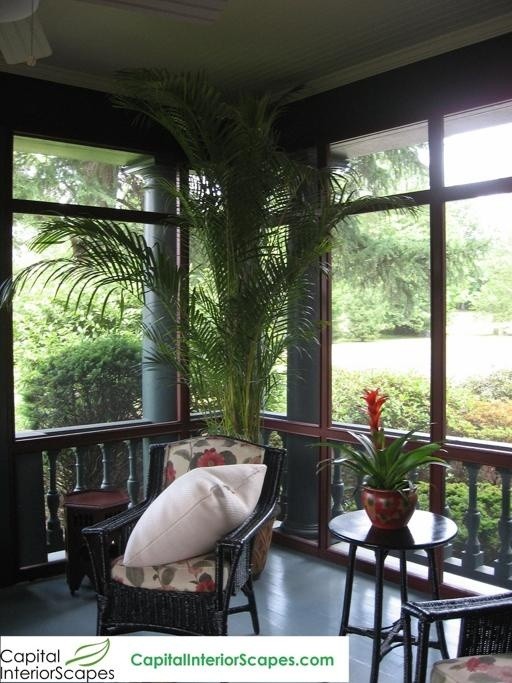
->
[305,386,455,503]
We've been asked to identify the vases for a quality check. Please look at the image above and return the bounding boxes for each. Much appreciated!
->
[359,479,419,530]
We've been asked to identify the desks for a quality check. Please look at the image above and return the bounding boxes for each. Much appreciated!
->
[60,488,132,596]
[327,508,459,683]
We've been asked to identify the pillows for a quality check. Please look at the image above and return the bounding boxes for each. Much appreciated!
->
[121,464,269,568]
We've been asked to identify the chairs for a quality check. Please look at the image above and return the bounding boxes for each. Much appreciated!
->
[400,591,511,682]
[81,436,290,635]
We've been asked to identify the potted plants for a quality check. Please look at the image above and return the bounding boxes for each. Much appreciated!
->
[0,64,420,580]
